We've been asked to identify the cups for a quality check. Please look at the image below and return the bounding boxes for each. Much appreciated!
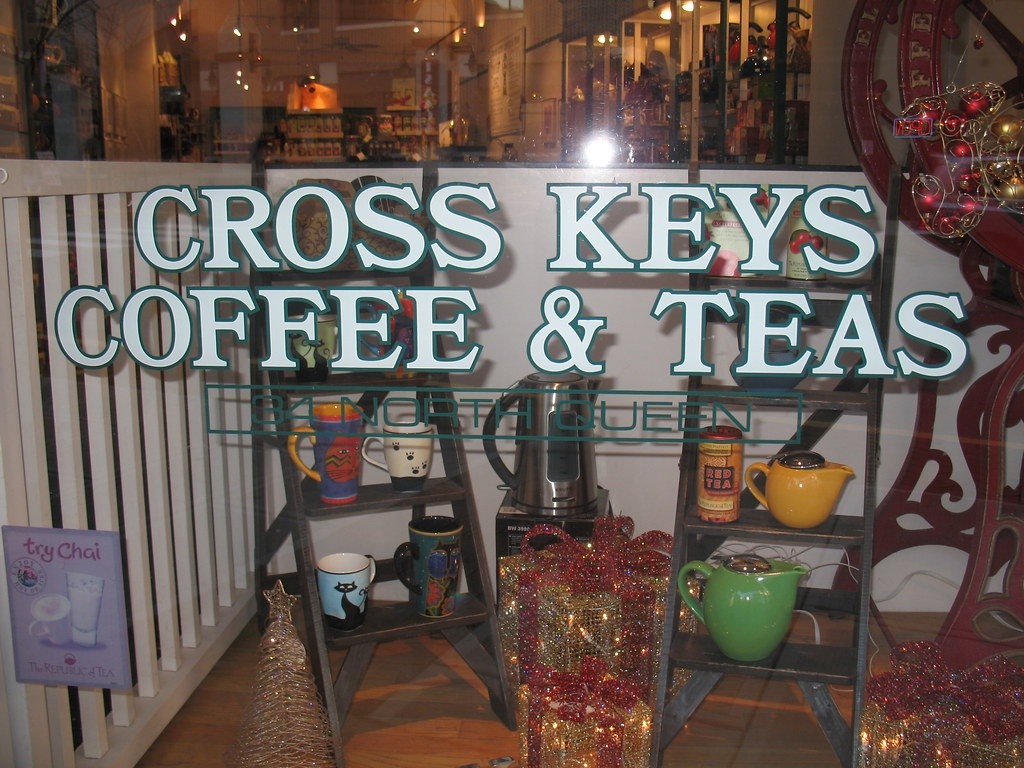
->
[285,314,337,383]
[360,293,414,380]
[362,421,434,494]
[67,572,104,648]
[288,402,364,504]
[317,552,377,632]
[394,516,464,617]
[28,592,73,647]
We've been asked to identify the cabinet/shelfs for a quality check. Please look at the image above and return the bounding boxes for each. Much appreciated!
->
[386,105,437,136]
[285,109,344,162]
[248,143,518,768]
[650,164,902,768]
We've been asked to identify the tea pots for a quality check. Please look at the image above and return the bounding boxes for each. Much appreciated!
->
[570,85,585,102]
[730,307,819,392]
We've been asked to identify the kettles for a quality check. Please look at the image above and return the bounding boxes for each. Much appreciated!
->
[483,370,604,517]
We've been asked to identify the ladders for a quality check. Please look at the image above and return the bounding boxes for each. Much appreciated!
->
[243,154,519,768]
[649,160,903,768]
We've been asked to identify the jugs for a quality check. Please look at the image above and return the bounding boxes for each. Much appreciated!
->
[745,449,855,528]
[677,554,809,662]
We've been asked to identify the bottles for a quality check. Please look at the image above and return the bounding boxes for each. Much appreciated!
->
[696,425,743,523]
[284,116,342,157]
[741,45,771,76]
[345,103,439,162]
